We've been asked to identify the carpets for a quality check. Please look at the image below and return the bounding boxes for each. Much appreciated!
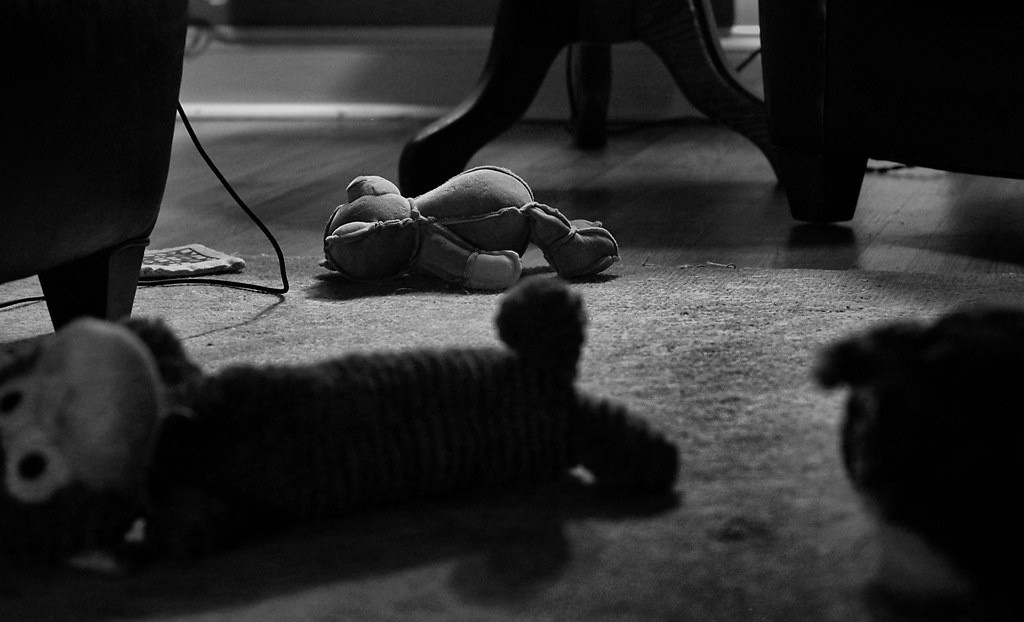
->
[0,264,1023,622]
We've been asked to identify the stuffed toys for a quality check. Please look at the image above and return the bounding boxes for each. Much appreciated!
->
[321,165,619,288]
[0,281,684,578]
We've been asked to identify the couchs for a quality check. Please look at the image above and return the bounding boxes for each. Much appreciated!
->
[0,0,191,335]
[759,0,1024,220]
